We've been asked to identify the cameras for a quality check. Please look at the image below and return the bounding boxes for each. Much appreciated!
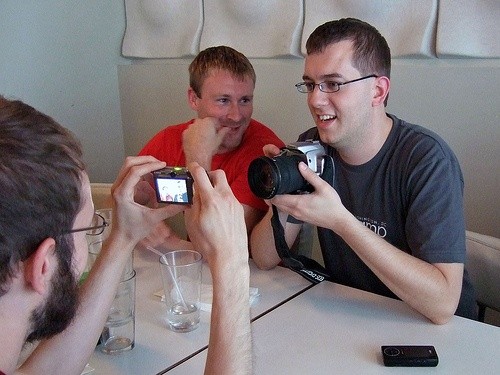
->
[152,166,209,205]
[382,345,439,367]
[248,138,335,199]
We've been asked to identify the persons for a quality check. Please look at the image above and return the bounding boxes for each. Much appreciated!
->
[0,94,253,375]
[133,46,301,268]
[250,17,480,325]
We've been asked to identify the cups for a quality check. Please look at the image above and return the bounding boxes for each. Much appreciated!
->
[95,208,112,239]
[87,237,134,273]
[100,269,137,355]
[158,249,203,334]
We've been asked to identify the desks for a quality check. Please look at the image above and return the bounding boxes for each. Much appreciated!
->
[18,248,500,375]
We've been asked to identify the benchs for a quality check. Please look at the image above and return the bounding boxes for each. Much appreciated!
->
[91,184,500,323]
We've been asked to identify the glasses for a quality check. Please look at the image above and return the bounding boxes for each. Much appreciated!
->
[19,213,109,263]
[295,74,380,93]
